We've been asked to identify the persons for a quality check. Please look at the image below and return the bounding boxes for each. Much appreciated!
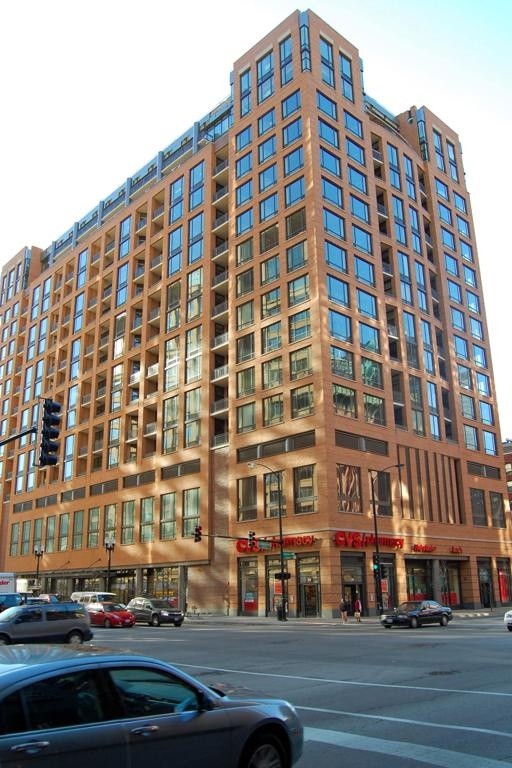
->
[276,598,288,621]
[339,598,349,624]
[354,597,362,622]
[81,673,149,721]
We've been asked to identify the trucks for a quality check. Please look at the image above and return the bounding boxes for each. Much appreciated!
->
[0,571,25,610]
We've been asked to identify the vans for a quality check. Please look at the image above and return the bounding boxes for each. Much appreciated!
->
[0,600,95,645]
[68,588,117,607]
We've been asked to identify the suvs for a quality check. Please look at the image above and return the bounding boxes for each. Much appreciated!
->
[125,596,185,628]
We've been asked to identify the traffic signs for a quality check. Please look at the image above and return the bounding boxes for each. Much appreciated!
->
[283,551,295,555]
[258,541,270,546]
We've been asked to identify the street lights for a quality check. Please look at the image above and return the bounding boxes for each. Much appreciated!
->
[247,459,291,621]
[100,534,117,592]
[32,542,45,596]
[367,462,406,620]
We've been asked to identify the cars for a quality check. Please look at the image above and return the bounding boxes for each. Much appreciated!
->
[0,641,306,767]
[86,598,136,629]
[21,591,68,605]
[378,599,453,628]
[502,608,512,629]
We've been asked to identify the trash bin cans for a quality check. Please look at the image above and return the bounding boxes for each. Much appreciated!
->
[277,607,286,621]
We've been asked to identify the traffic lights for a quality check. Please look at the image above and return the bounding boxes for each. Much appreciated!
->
[284,573,290,579]
[380,563,388,579]
[36,396,65,467]
[372,552,380,579]
[247,527,257,548]
[275,572,282,578]
[192,524,204,542]
